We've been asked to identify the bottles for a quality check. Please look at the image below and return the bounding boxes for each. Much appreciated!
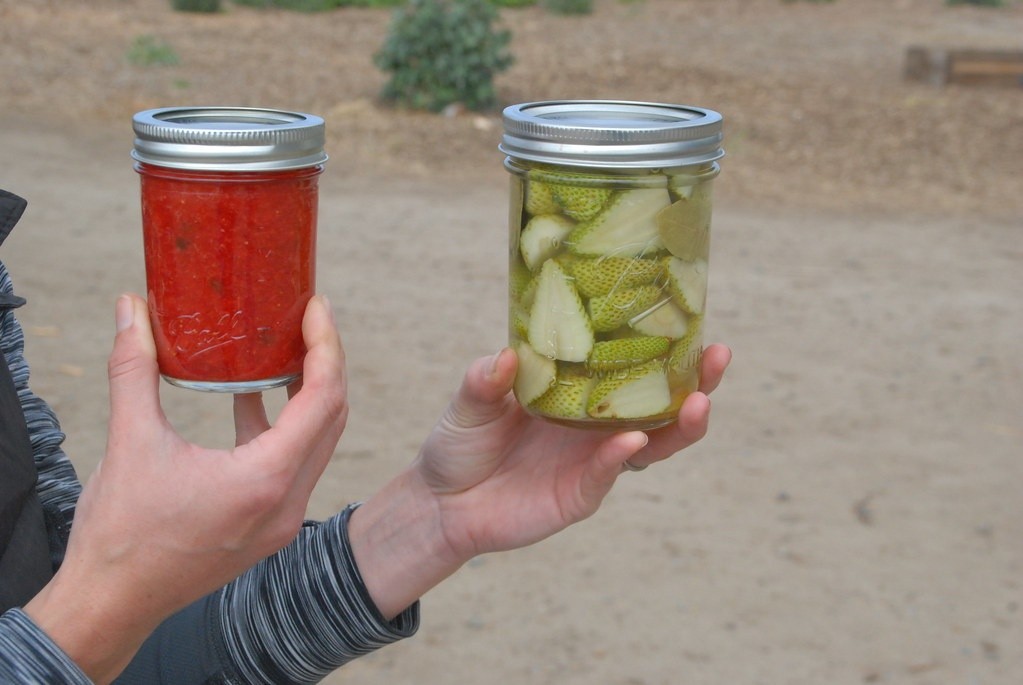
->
[500,99,723,430]
[134,107,325,396]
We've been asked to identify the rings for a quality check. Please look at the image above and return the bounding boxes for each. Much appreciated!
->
[624,461,646,472]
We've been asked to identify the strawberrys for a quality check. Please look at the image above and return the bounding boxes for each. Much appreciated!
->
[509,165,708,421]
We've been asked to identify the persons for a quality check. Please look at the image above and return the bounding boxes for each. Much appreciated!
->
[0,190,732,685]
